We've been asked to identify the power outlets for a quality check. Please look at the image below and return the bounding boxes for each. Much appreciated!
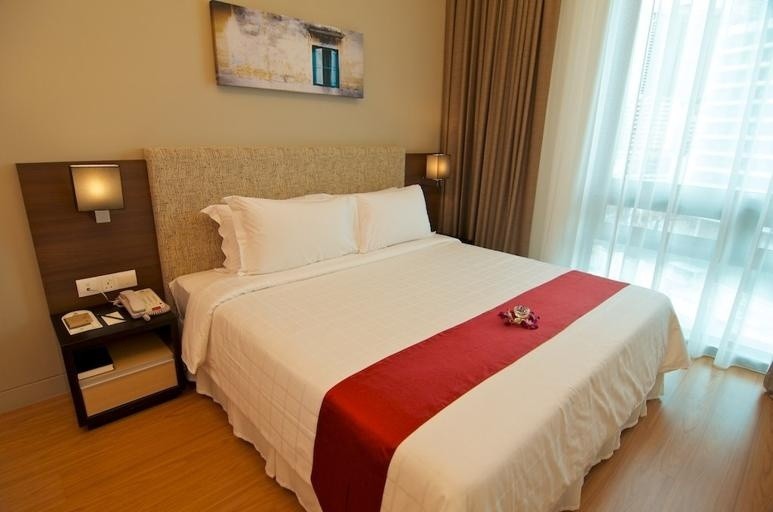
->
[74,270,138,298]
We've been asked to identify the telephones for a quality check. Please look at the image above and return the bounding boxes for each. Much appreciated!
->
[114,288,170,321]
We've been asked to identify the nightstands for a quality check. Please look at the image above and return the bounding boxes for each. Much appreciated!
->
[51,291,187,429]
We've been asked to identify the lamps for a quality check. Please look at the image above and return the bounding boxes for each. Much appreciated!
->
[420,154,451,189]
[71,164,125,223]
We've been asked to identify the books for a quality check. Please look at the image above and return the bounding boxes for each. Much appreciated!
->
[75,344,115,380]
[65,313,94,329]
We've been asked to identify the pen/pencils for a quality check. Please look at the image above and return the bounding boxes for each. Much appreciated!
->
[97,314,127,320]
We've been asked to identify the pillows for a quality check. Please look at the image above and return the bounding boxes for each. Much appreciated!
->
[199,192,333,272]
[227,195,355,274]
[351,185,431,253]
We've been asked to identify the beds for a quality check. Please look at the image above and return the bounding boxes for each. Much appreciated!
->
[143,144,689,512]
